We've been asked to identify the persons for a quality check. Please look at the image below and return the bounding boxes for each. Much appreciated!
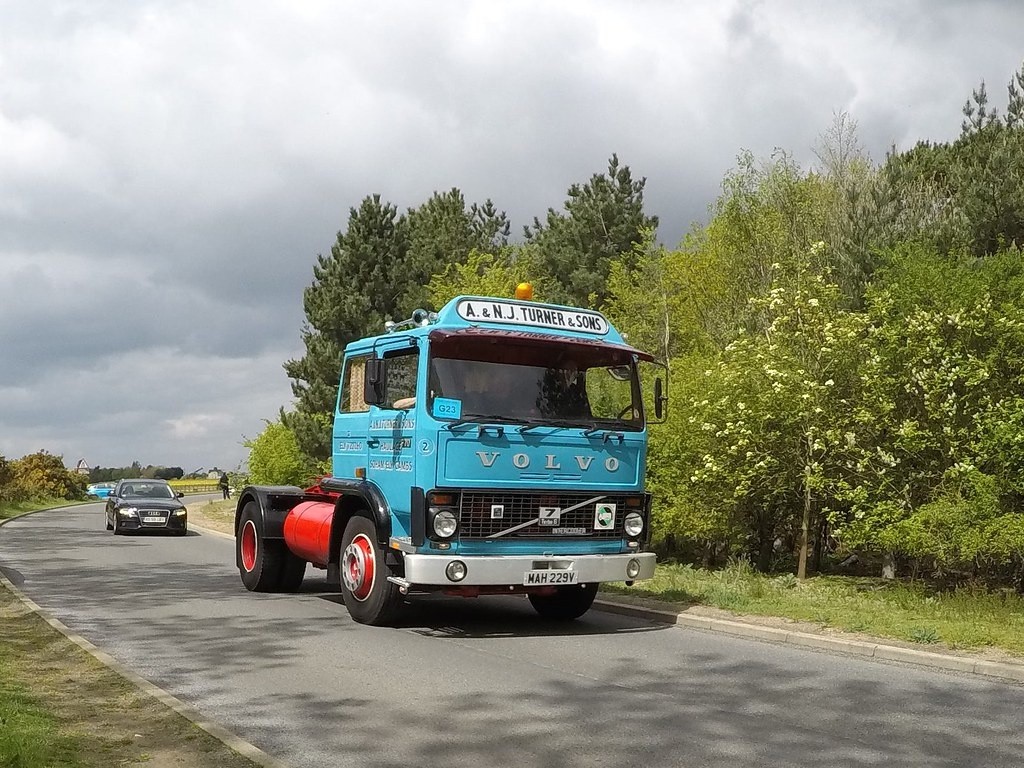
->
[392,390,434,408]
[464,371,537,418]
[220,473,230,499]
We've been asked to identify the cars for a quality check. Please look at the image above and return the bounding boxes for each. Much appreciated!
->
[87,484,114,497]
[104,478,189,535]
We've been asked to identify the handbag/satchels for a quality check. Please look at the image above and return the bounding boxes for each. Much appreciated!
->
[220,482,228,489]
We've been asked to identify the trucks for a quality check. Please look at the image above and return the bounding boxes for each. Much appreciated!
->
[232,295,668,627]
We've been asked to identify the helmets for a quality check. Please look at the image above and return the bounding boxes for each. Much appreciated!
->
[554,358,577,381]
